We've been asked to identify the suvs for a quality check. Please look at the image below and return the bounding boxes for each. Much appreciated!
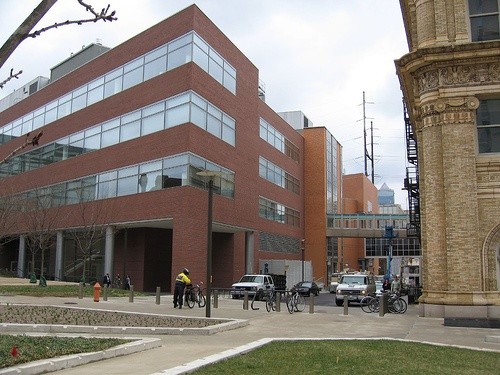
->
[336,274,376,306]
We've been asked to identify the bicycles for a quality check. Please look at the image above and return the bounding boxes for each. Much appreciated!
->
[360,290,407,314]
[265,287,276,312]
[287,285,305,314]
[186,281,206,308]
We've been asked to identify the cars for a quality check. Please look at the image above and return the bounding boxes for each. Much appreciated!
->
[290,281,320,298]
[230,275,275,299]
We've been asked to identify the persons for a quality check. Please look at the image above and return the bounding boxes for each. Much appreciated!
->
[174,269,191,308]
[105,272,110,287]
[382,279,391,291]
[391,276,401,293]
[116,274,120,286]
[126,275,131,289]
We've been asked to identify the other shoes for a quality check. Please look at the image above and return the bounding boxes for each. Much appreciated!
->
[174,304,178,308]
[179,305,182,309]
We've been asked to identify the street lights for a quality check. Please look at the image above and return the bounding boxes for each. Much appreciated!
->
[196,171,222,318]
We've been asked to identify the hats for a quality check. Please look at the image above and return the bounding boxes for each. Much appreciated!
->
[184,268,190,275]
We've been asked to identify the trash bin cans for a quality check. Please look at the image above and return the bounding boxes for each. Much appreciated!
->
[407,286,422,305]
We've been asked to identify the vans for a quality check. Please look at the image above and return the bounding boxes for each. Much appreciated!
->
[329,273,400,299]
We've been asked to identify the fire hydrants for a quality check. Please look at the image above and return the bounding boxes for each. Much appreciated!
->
[93,282,102,302]
